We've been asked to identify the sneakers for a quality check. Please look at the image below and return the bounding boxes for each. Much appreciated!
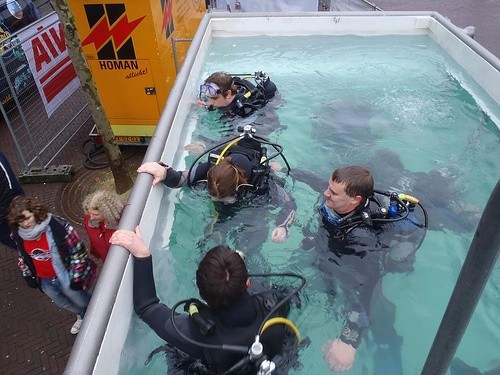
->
[70,315,83,334]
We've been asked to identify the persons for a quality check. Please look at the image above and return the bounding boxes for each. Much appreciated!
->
[7,196,92,335]
[109,225,308,375]
[136,144,298,261]
[183,71,280,155]
[82,191,123,263]
[270,161,381,371]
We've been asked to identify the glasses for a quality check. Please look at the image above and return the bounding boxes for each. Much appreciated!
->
[200,83,221,98]
[211,189,238,204]
[321,202,356,227]
